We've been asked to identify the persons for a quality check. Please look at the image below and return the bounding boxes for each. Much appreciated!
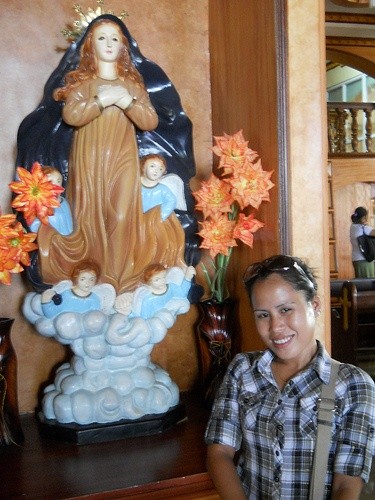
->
[206,255,375,500]
[350,207,375,279]
[35,15,197,322]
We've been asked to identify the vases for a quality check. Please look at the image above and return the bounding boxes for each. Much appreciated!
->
[195,300,234,416]
[0,316,24,446]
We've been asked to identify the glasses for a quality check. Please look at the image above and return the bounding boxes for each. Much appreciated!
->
[241,255,314,295]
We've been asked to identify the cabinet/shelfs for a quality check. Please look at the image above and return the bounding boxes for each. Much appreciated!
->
[0,0,287,500]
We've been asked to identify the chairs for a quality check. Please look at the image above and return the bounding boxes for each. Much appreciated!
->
[330,278,375,366]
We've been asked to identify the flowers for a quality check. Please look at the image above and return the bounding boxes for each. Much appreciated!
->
[191,129,276,302]
[0,162,65,286]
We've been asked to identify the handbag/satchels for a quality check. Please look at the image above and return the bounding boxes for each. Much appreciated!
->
[357,224,375,262]
